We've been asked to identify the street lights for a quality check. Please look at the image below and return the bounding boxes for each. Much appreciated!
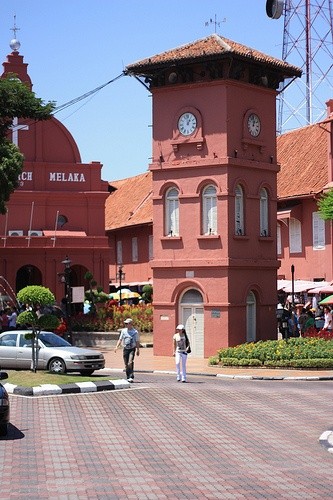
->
[115,265,125,308]
[57,256,74,320]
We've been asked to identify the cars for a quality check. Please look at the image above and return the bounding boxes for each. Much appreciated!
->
[0,329,105,377]
[0,371,10,438]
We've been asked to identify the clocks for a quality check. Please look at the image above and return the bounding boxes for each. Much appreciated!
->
[177,112,197,136]
[247,113,261,137]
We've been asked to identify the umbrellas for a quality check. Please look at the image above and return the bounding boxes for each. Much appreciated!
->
[318,295,333,305]
[109,289,141,301]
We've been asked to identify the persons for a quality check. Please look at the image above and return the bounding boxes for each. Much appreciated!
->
[114,318,140,383]
[277,295,333,340]
[0,303,65,336]
[83,297,147,315]
[173,325,190,382]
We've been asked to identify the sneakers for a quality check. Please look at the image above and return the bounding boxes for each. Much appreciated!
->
[177,375,180,381]
[127,378,133,383]
[182,376,186,382]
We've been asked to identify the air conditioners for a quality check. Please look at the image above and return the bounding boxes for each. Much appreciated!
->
[8,230,43,237]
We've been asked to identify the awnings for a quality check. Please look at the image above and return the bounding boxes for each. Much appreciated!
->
[277,279,333,294]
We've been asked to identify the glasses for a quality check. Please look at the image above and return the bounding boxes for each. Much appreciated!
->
[125,323,131,324]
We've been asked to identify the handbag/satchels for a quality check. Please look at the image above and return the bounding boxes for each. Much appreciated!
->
[185,333,191,353]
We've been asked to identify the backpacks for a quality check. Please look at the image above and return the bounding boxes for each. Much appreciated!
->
[122,331,137,350]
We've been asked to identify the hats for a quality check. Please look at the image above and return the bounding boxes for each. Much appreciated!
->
[124,319,133,323]
[176,325,185,330]
